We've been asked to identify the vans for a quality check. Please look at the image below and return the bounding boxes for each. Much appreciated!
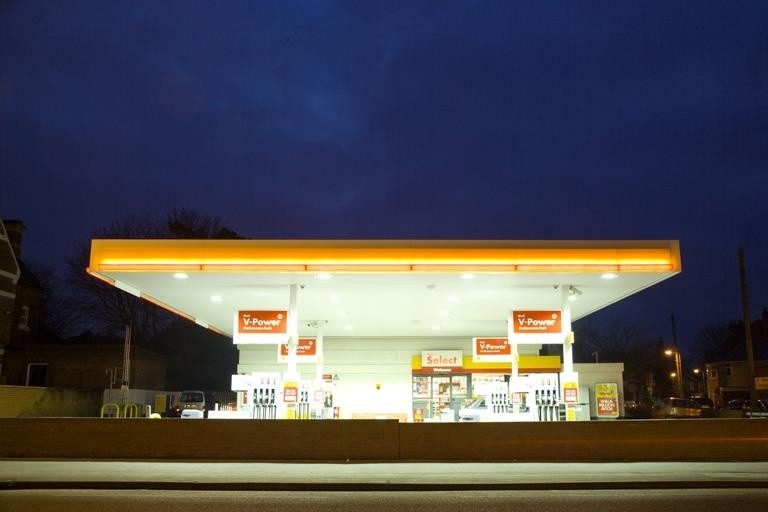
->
[176,390,221,418]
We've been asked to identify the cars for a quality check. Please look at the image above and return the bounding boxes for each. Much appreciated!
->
[625,395,714,420]
[727,397,768,419]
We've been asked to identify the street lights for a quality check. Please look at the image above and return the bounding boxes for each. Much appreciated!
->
[693,368,706,396]
[664,348,683,397]
[670,372,682,396]
